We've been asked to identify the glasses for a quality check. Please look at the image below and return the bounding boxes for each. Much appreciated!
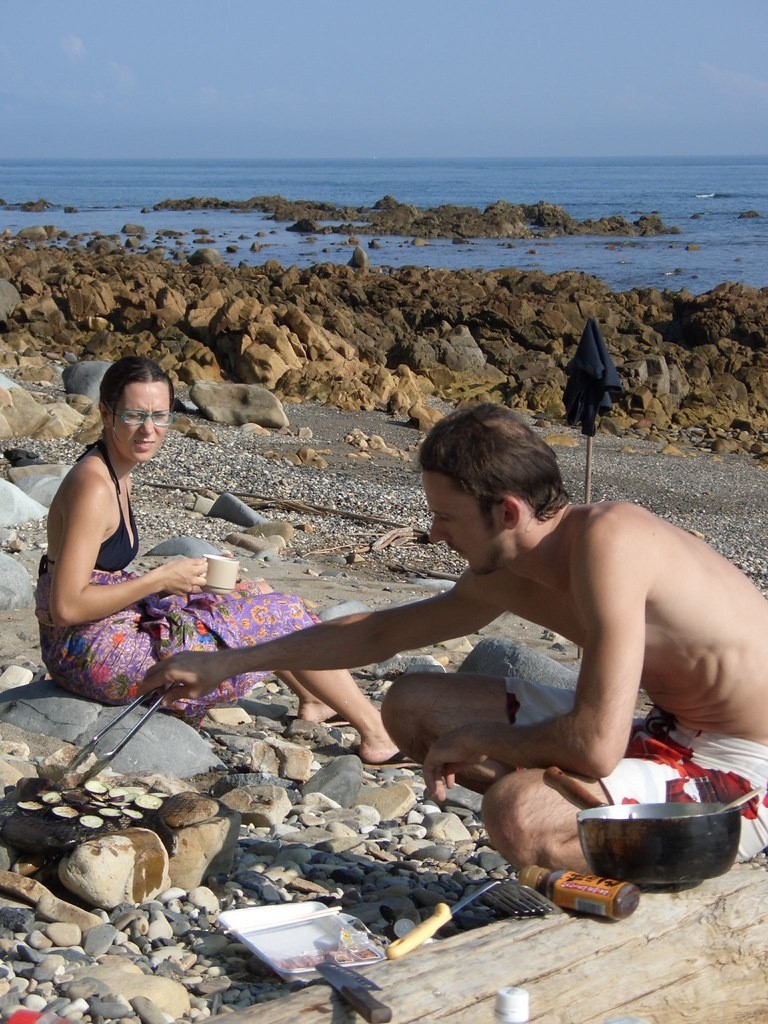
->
[103,399,174,426]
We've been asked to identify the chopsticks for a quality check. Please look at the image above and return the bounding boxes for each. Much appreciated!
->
[224,905,343,933]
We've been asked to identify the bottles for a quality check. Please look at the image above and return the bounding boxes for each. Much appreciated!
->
[517,865,642,922]
[489,988,530,1024]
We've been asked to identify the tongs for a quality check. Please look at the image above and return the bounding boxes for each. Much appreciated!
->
[63,677,172,786]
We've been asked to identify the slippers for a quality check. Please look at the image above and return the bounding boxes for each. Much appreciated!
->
[362,750,423,769]
[317,714,350,727]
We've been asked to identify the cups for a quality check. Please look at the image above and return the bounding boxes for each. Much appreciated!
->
[200,552,240,595]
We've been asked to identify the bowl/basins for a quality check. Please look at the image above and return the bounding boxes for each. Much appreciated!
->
[576,803,743,892]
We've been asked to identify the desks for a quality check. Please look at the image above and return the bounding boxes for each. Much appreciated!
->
[184,862,768,1024]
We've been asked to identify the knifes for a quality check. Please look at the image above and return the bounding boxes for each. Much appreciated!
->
[314,960,392,1023]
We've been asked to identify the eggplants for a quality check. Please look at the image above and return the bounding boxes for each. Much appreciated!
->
[17,782,170,829]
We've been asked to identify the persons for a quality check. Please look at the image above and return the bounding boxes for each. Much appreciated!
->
[136,400,767,909]
[35,350,422,765]
